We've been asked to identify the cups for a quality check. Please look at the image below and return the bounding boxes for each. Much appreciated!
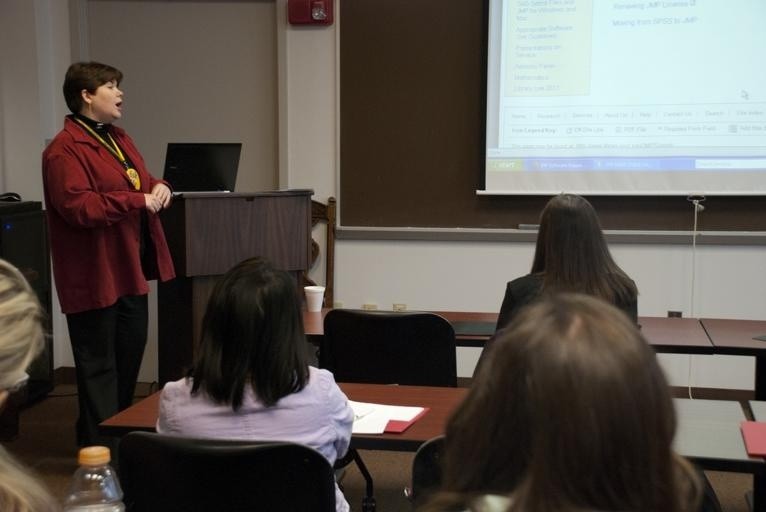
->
[302,285,327,313]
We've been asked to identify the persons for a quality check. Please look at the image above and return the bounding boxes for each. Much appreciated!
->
[1,260,71,511]
[393,289,724,511]
[41,62,178,444]
[496,193,639,327]
[151,255,355,511]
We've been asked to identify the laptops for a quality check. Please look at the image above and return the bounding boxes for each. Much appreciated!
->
[163,143,242,192]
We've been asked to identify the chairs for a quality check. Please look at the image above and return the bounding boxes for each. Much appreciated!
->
[302,196,338,311]
[119,308,766,512]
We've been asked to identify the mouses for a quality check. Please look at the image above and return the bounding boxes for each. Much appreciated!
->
[1,192,21,201]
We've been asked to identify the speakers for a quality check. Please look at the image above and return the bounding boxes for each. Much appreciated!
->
[0,201,55,408]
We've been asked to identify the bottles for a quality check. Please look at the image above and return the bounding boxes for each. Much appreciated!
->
[66,446,124,510]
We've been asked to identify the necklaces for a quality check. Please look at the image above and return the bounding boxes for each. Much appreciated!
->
[74,117,141,191]
